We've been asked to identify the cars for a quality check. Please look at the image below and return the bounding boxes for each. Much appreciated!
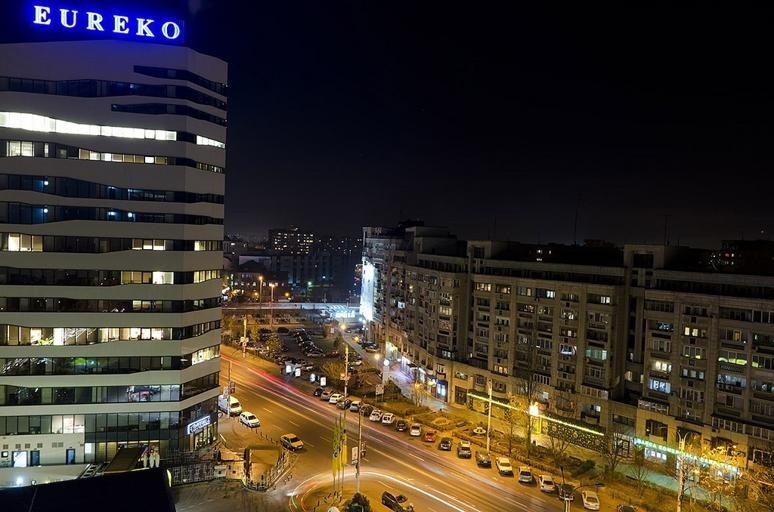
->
[280,432,304,451]
[229,327,326,371]
[240,411,260,428]
[328,333,376,371]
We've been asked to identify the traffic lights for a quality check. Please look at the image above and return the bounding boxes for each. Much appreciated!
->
[229,381,235,393]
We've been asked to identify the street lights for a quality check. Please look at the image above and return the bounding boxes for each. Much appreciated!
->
[732,445,749,471]
[645,426,699,512]
[285,290,292,302]
[259,275,263,301]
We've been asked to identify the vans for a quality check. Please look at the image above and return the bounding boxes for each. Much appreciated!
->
[218,394,242,417]
